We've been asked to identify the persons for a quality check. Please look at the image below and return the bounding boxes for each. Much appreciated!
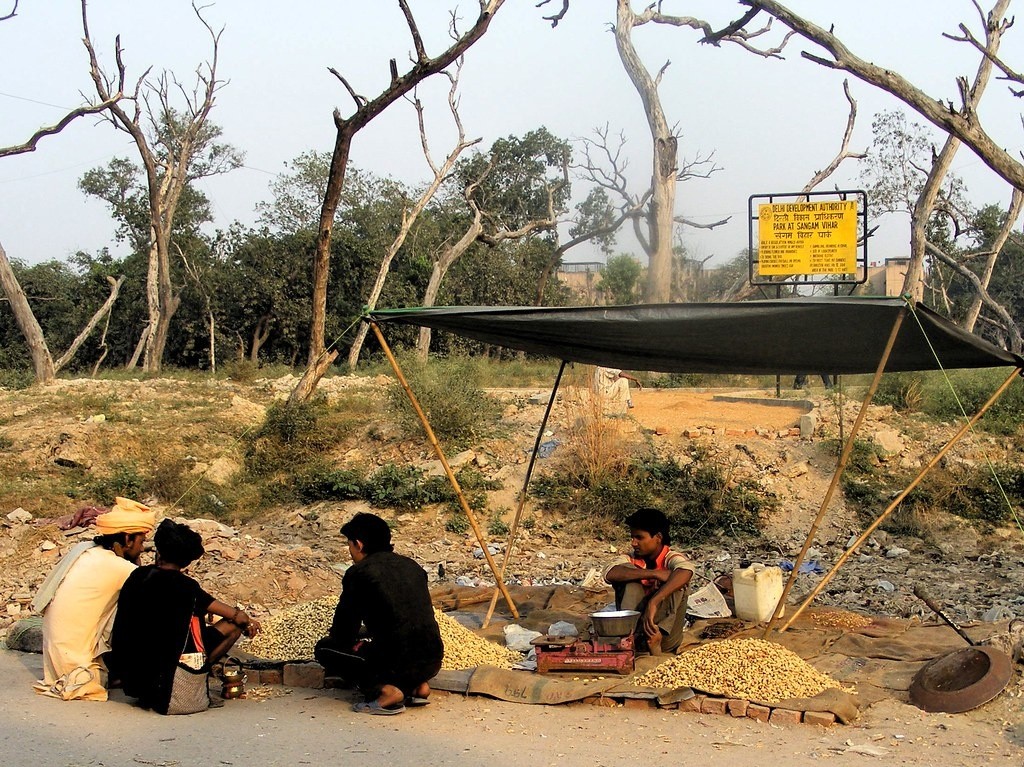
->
[35,496,155,701]
[112,519,263,714]
[594,366,643,408]
[315,512,443,717]
[605,508,695,654]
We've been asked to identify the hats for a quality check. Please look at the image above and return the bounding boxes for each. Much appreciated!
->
[96,497,154,534]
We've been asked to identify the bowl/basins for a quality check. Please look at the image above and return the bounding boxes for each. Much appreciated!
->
[587,610,641,637]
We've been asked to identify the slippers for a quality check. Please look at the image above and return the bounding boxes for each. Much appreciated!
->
[351,699,406,715]
[406,696,430,703]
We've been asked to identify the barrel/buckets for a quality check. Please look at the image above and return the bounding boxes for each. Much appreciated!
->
[733,563,785,623]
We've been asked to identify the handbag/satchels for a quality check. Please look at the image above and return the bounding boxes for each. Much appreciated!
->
[51,663,106,701]
[165,659,209,714]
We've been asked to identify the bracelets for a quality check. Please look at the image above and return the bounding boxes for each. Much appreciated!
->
[228,607,240,622]
[245,620,253,629]
